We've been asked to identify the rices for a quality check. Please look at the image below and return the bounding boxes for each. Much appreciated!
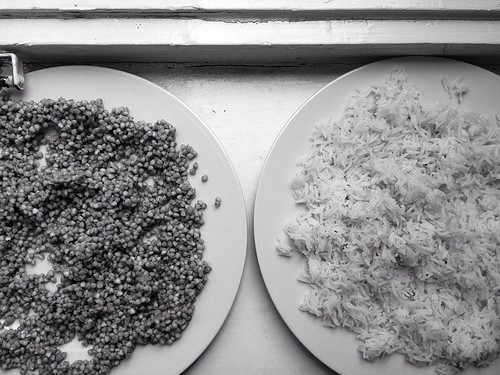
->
[273,69,500,375]
